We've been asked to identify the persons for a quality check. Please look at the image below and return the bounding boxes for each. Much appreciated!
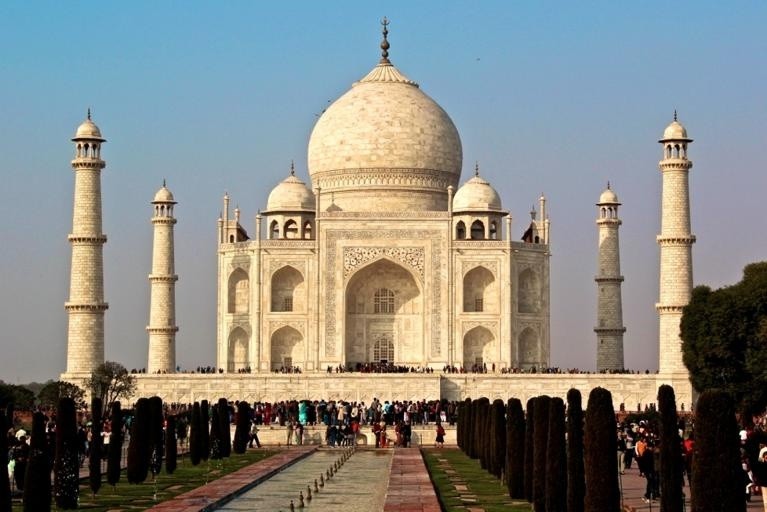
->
[8,399,766,511]
[128,362,660,374]
